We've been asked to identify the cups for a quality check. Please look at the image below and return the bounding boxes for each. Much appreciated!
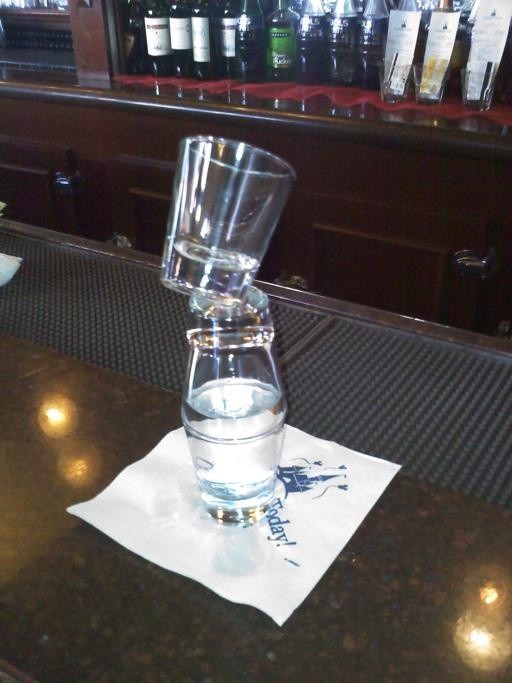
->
[381,53,498,111]
[160,137,297,304]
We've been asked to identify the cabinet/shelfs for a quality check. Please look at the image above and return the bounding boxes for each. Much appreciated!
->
[1,0,121,88]
[0,86,105,244]
[105,97,277,286]
[277,117,512,341]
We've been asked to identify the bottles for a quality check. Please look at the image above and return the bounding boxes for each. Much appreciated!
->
[179,285,287,527]
[120,0,273,83]
[265,0,423,90]
[425,0,463,90]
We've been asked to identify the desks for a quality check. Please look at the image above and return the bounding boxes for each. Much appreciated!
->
[0,215,512,683]
